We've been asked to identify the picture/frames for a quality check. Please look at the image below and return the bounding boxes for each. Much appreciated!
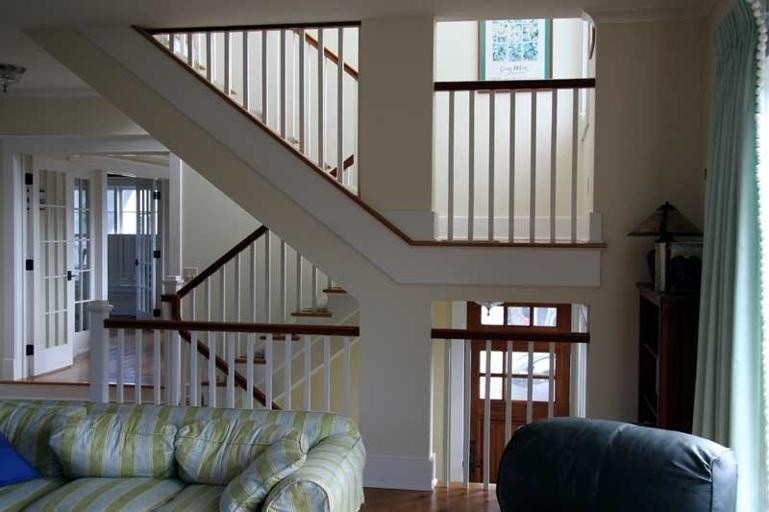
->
[476,17,554,94]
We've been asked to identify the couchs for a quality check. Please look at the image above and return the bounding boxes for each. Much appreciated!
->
[0,398,369,511]
[495,415,740,512]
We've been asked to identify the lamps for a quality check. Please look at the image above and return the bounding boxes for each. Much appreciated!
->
[627,199,703,294]
[0,62,27,96]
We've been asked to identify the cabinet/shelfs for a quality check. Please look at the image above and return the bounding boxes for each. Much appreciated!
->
[634,281,699,434]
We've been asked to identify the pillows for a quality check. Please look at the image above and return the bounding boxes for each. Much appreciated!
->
[46,411,178,481]
[218,431,310,512]
[173,417,297,486]
[0,401,90,480]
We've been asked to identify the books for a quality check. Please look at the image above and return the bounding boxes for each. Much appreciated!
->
[653,240,702,296]
